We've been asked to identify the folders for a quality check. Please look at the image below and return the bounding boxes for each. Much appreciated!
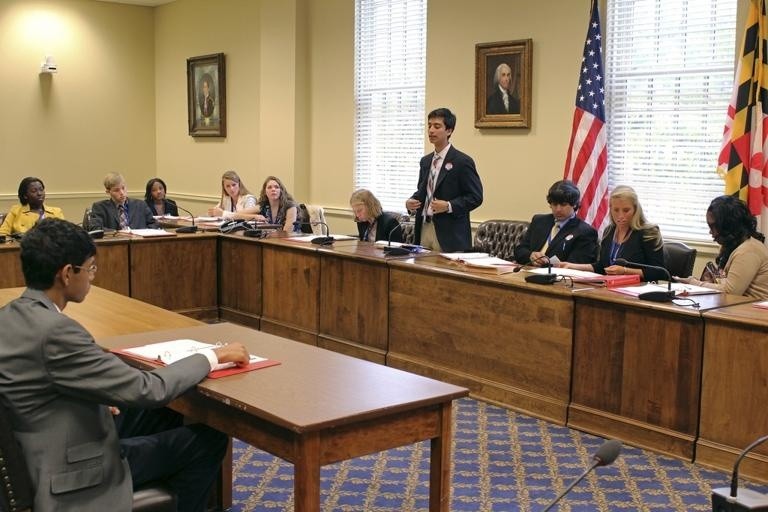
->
[572,275,640,287]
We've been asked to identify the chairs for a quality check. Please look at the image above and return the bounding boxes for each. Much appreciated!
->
[295,202,327,237]
[380,206,412,226]
[661,237,698,285]
[399,223,425,245]
[0,407,184,512]
[468,218,534,262]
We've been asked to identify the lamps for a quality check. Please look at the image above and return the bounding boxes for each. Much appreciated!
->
[39,55,58,73]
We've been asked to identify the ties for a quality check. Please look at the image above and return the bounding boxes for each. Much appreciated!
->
[423,155,441,224]
[540,222,558,257]
[118,204,129,229]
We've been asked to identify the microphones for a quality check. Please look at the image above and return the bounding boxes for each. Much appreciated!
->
[541,440,622,512]
[513,256,557,285]
[712,434,768,512]
[292,221,334,245]
[84,218,104,239]
[384,210,418,255]
[165,199,198,233]
[244,218,266,237]
[616,257,675,302]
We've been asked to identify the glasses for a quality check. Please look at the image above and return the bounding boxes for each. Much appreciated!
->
[73,264,98,274]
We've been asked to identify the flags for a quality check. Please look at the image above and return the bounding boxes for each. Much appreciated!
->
[717,1,768,219]
[563,1,609,238]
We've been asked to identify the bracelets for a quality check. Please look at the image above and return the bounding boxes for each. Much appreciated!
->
[623,266,626,275]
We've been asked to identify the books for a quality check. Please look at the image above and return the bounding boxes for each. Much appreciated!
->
[438,249,519,268]
[375,236,431,253]
[284,234,356,243]
[117,228,177,238]
[526,265,722,297]
[152,213,270,227]
[751,300,768,311]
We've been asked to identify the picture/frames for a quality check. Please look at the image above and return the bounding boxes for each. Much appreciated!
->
[474,38,531,130]
[186,52,227,139]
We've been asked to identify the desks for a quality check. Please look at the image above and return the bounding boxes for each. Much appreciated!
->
[1,282,213,347]
[104,320,471,511]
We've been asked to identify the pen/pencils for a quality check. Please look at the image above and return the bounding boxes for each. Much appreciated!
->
[491,264,518,266]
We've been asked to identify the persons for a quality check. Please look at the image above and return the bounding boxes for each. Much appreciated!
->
[671,195,768,300]
[144,179,181,220]
[406,108,484,253]
[0,216,252,511]
[551,186,671,283]
[199,78,214,117]
[231,176,302,233]
[209,170,257,215]
[487,64,519,114]
[514,180,596,271]
[349,188,404,243]
[89,172,161,234]
[0,178,68,236]
